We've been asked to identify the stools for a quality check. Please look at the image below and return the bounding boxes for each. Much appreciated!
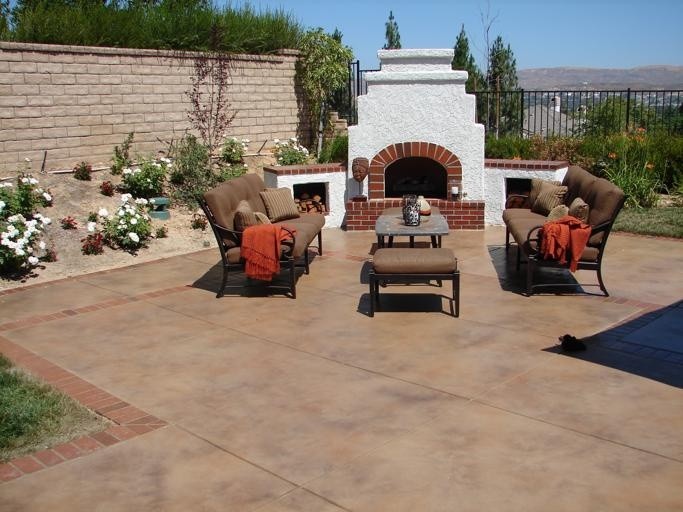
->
[367,248,460,317]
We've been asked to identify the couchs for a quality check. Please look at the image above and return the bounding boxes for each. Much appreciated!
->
[194,172,325,299]
[502,165,630,297]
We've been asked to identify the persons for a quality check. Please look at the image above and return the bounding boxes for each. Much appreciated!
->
[352,157,370,182]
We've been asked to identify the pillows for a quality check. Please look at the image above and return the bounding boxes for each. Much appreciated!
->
[529,178,590,226]
[234,187,301,240]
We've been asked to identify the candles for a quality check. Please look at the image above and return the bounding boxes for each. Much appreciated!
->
[451,185,458,195]
